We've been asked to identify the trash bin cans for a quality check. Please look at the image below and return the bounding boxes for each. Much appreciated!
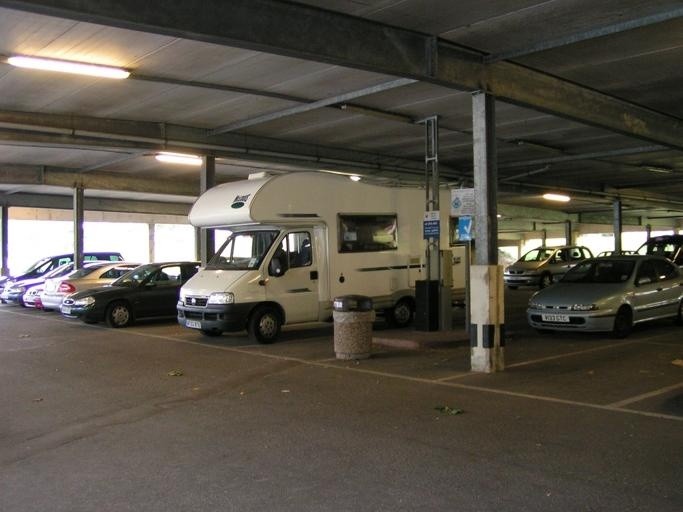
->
[334,295,375,362]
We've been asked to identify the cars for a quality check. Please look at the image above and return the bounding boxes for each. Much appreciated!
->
[504,234,683,339]
[0,252,202,328]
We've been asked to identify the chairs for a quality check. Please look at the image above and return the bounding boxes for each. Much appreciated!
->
[593,263,621,281]
[272,240,311,266]
[158,273,170,282]
[543,252,579,262]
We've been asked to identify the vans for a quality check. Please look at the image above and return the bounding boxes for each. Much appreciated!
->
[176,170,467,344]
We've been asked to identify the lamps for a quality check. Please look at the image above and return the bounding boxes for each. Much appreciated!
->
[6,55,133,83]
